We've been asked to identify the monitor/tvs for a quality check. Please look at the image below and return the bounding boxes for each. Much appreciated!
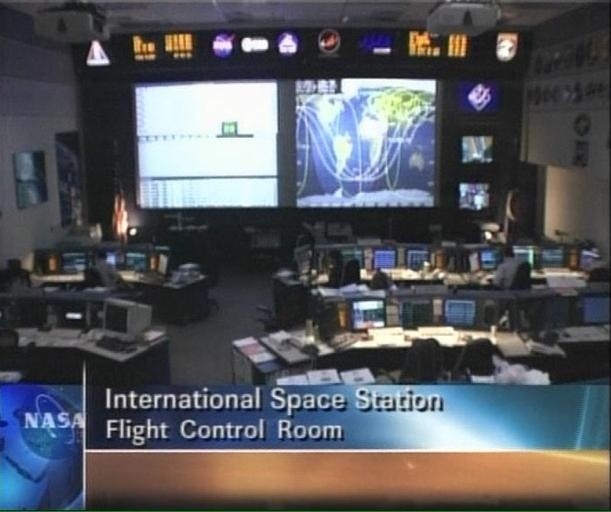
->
[289,238,601,273]
[37,248,175,282]
[5,298,157,339]
[317,297,610,342]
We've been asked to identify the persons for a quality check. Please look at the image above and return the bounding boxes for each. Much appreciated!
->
[0,210,611,390]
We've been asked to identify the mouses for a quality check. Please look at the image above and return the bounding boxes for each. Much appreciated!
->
[126,345,137,353]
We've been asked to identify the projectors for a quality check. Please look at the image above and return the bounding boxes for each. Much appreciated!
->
[31,7,111,46]
[427,0,501,35]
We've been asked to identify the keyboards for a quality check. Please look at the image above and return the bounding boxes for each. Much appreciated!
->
[97,335,125,352]
